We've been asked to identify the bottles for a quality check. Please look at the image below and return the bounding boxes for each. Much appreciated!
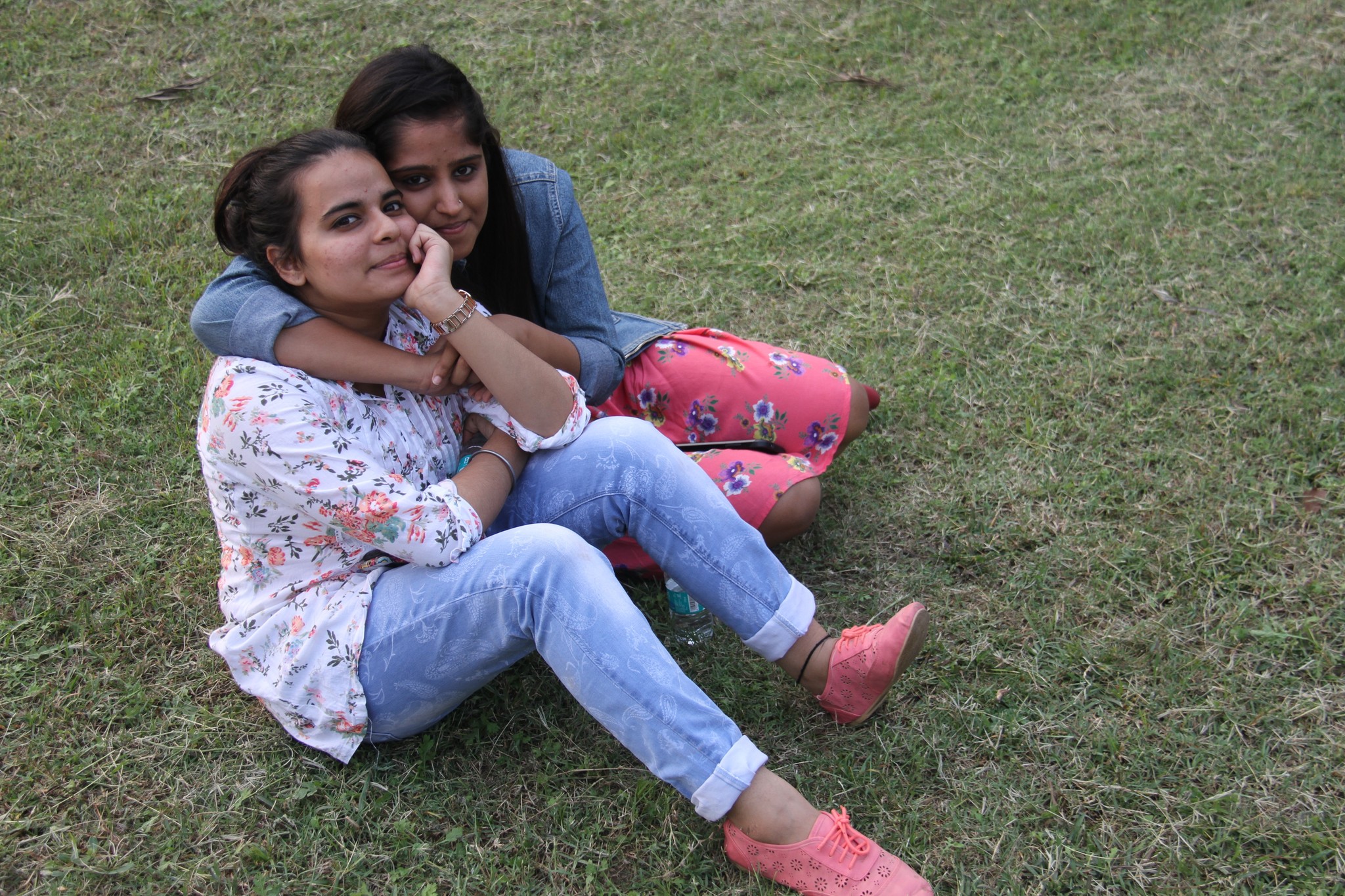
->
[662,568,713,645]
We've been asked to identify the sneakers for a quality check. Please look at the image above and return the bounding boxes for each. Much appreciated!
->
[812,601,930,726]
[724,803,934,896]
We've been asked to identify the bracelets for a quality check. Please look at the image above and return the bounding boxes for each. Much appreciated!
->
[468,447,515,496]
[432,287,476,338]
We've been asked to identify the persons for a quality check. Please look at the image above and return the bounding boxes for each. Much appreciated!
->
[195,131,935,896]
[189,44,881,575]
